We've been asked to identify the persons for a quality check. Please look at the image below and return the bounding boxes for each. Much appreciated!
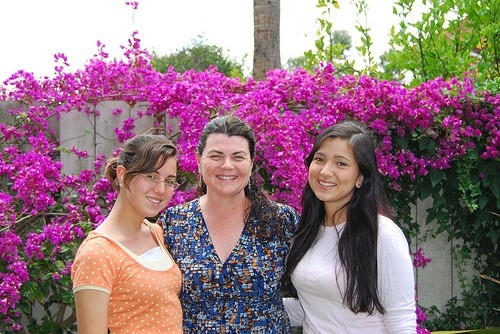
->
[156,116,301,334]
[71,133,184,334]
[278,123,418,334]
